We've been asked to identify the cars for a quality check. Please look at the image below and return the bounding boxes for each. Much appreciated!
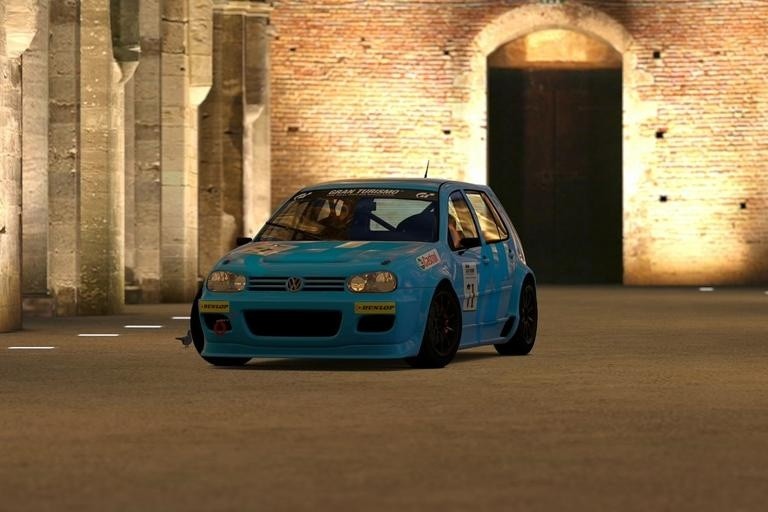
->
[190,160,539,368]
